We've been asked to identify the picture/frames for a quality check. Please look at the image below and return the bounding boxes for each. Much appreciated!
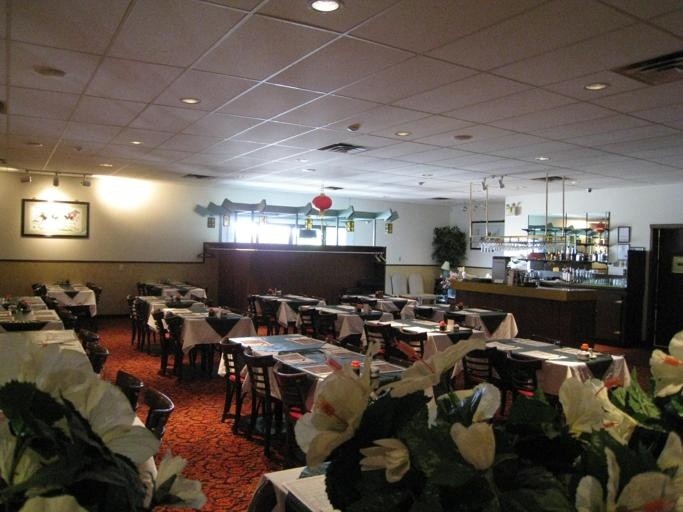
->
[618,226,632,244]
[20,199,91,241]
[469,220,504,251]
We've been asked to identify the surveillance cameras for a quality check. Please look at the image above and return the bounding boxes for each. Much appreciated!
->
[587,188,592,192]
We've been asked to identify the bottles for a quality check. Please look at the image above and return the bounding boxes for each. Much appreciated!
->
[548,246,608,284]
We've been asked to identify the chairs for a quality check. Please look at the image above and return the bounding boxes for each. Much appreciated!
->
[421,292,629,406]
[125,280,418,381]
[32,281,47,296]
[60,308,78,328]
[85,280,103,332]
[139,387,177,441]
[271,364,309,465]
[43,295,60,310]
[87,343,110,373]
[241,345,274,456]
[114,369,144,412]
[78,329,100,351]
[219,336,244,434]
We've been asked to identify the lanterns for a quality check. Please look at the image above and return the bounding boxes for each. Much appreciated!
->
[311,193,332,216]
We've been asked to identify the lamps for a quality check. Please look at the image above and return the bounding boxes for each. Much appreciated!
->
[384,220,395,235]
[77,174,93,192]
[305,217,313,229]
[22,170,33,187]
[223,215,231,227]
[346,220,356,232]
[442,259,452,279]
[51,171,60,190]
[207,215,216,228]
